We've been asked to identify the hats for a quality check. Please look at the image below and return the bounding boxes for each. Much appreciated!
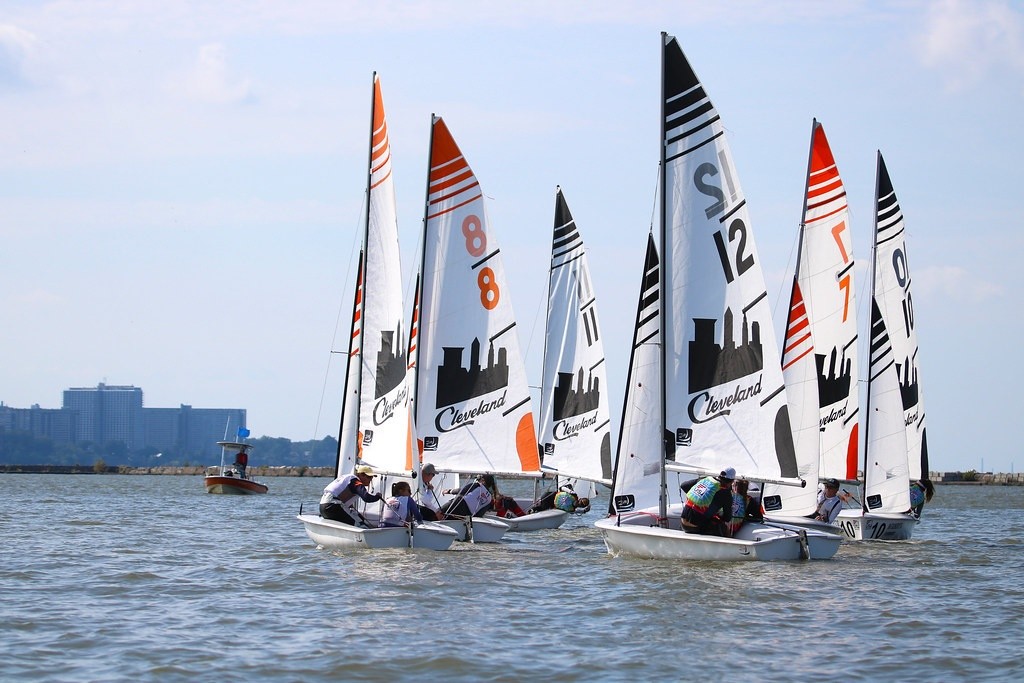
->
[356,466,377,477]
[823,479,839,488]
[422,463,439,474]
[720,468,737,480]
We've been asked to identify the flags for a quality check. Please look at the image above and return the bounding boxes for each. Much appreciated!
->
[238,426,250,437]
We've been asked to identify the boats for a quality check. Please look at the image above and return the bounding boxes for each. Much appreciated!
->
[203,438,269,494]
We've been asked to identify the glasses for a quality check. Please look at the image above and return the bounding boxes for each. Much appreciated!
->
[431,473,435,476]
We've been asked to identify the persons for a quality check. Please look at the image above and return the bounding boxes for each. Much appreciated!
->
[900,479,935,518]
[434,473,495,521]
[379,482,423,528]
[319,466,383,529]
[680,467,736,538]
[488,472,526,518]
[234,446,248,479]
[527,492,589,515]
[803,479,842,525]
[717,477,763,538]
[409,463,443,522]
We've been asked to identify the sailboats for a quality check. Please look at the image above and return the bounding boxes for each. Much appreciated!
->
[294,22,938,564]
